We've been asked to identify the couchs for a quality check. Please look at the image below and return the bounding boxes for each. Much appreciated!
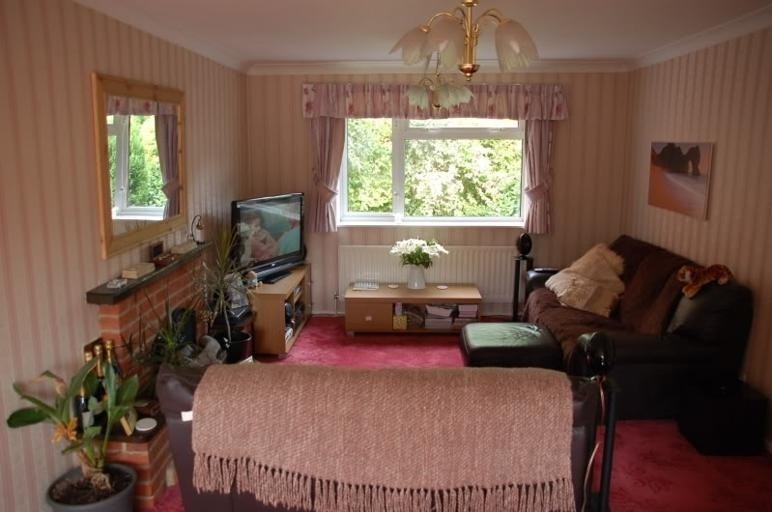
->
[154,352,602,512]
[522,233,756,424]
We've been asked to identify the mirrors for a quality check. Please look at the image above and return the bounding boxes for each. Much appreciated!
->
[88,69,192,261]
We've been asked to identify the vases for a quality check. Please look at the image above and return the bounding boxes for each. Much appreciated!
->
[43,460,140,511]
[406,264,426,291]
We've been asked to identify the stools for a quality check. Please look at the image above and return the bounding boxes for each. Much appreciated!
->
[457,319,564,371]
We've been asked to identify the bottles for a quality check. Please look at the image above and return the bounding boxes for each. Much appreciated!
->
[73,338,123,436]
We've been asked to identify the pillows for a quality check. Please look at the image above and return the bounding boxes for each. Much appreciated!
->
[570,245,626,275]
[543,251,628,311]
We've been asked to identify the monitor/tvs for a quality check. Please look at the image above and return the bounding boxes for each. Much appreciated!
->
[231,191,307,287]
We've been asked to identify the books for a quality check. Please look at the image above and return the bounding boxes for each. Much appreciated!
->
[426,304,457,316]
[425,314,452,328]
[453,304,477,326]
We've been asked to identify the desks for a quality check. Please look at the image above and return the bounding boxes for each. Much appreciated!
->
[342,281,484,337]
[671,378,771,459]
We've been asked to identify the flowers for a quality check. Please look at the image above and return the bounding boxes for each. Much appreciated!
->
[6,352,143,490]
[388,236,451,270]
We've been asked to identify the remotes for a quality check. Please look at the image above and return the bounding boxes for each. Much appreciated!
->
[534,267,560,273]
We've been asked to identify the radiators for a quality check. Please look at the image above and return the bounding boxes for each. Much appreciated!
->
[335,242,530,307]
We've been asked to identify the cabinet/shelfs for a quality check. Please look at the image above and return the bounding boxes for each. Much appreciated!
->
[246,259,314,361]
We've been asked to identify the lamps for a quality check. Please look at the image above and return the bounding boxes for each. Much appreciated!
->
[383,0,547,116]
[188,212,207,247]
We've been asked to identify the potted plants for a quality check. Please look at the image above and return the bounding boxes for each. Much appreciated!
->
[195,227,256,365]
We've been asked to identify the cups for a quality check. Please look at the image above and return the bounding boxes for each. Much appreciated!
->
[248,273,258,287]
